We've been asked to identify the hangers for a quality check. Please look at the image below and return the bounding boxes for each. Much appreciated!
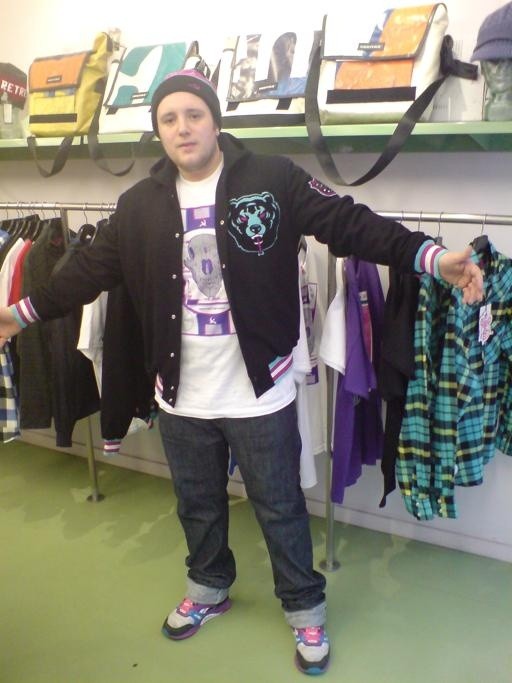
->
[0,202,115,246]
[471,213,492,254]
[434,211,442,246]
[416,212,424,232]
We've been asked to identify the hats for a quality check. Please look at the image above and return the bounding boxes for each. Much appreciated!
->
[470,2,512,62]
[0,63,27,109]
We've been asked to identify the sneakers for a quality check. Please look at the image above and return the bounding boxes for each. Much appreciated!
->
[162,598,232,639]
[294,625,332,675]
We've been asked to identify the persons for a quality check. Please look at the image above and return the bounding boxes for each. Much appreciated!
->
[0,68,485,675]
